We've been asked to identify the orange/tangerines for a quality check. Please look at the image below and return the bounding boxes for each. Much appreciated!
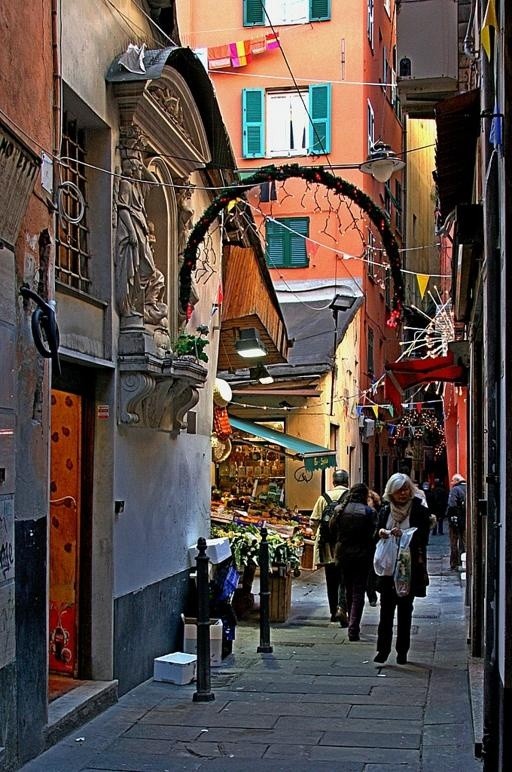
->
[239,517,258,523]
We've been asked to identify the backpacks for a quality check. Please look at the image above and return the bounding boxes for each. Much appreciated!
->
[319,490,349,541]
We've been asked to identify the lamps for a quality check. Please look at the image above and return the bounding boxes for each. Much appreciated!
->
[221,325,274,384]
[357,0,407,184]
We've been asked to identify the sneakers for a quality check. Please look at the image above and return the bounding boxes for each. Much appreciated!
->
[348,622,361,641]
[372,647,391,668]
[395,651,408,664]
[334,605,349,627]
[330,608,340,622]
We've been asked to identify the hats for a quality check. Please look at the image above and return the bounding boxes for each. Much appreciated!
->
[333,469,349,478]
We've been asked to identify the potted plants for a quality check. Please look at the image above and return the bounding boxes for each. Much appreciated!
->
[176,334,209,365]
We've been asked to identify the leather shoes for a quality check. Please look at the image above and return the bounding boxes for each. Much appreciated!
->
[370,600,376,606]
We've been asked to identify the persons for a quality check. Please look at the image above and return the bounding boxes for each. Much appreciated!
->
[326,482,380,643]
[399,465,449,537]
[366,489,382,608]
[307,469,351,628]
[363,472,432,665]
[112,149,157,318]
[143,219,172,331]
[443,473,468,572]
